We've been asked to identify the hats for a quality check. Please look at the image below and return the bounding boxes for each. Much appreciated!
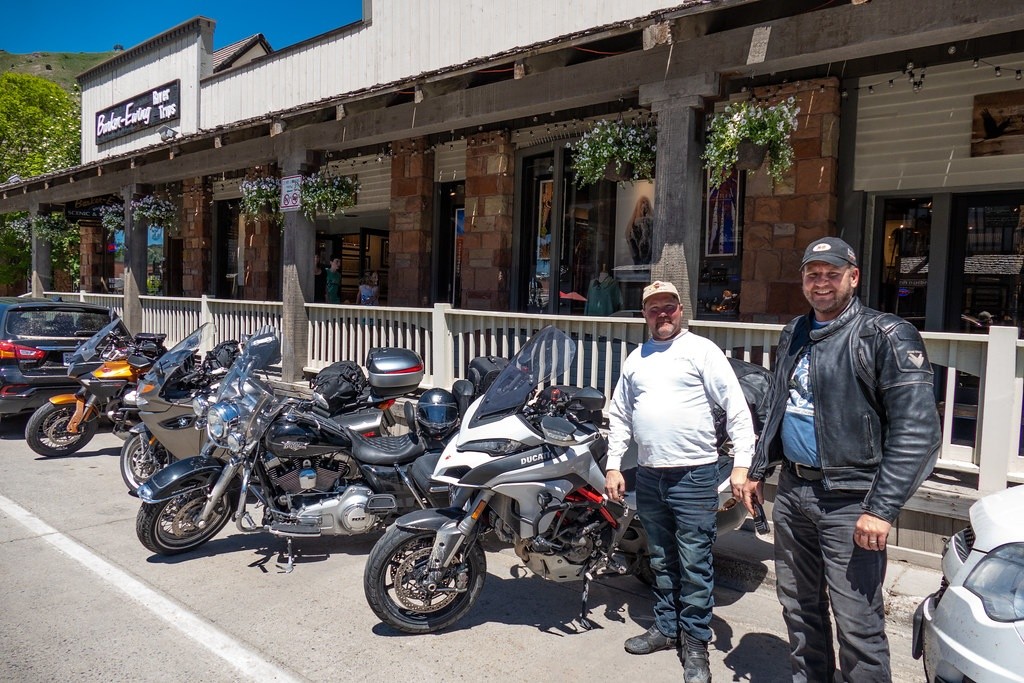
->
[799,237,860,272]
[642,280,680,311]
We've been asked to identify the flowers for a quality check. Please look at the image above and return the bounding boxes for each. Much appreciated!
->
[98,204,125,241]
[697,96,801,191]
[129,195,181,238]
[564,118,659,192]
[297,170,362,222]
[238,176,285,234]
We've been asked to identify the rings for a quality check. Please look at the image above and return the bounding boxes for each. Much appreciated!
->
[869,541,876,544]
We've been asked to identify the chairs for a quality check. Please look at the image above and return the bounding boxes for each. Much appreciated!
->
[11,317,42,336]
[76,315,105,331]
[100,277,124,294]
[53,313,74,333]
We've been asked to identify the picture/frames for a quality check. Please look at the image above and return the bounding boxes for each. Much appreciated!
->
[381,239,390,269]
[342,234,370,252]
[371,270,387,301]
[341,254,371,278]
[340,285,361,304]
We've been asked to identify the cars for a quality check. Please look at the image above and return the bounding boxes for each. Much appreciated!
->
[0,295,124,429]
[912,484,1024,683]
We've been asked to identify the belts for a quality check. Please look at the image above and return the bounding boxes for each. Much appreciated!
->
[782,458,823,482]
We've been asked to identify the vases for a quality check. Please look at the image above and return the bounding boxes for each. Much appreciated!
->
[603,157,634,182]
[115,223,124,230]
[259,201,273,215]
[732,137,771,171]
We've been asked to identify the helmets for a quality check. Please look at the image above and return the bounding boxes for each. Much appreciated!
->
[416,388,458,441]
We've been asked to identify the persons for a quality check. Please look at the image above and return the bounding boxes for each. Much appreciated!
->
[743,236,941,683]
[719,290,732,310]
[605,281,756,683]
[314,254,329,303]
[324,254,349,304]
[356,271,380,306]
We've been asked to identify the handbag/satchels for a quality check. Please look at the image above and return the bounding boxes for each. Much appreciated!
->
[204,340,239,368]
[727,357,775,430]
[310,360,366,415]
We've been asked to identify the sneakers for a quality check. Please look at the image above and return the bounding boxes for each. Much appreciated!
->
[624,622,679,654]
[680,628,712,683]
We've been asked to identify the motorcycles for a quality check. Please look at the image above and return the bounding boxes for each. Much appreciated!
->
[25,315,283,456]
[134,327,511,574]
[363,321,778,631]
[113,322,426,495]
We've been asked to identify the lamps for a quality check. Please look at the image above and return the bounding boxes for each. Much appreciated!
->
[8,174,20,183]
[156,125,179,142]
